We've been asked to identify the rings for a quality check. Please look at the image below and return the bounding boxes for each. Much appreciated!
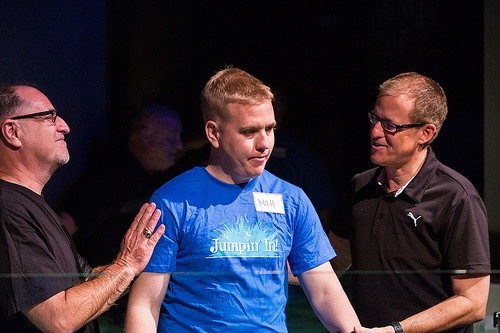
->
[144,231,152,238]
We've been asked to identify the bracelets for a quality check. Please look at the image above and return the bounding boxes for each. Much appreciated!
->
[386,322,403,333]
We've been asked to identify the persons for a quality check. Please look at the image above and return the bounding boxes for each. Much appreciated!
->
[57,74,336,264]
[288,71,490,333]
[125,69,362,333]
[0,82,165,333]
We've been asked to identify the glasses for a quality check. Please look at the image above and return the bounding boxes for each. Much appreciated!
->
[7,109,57,123]
[367,110,425,135]
